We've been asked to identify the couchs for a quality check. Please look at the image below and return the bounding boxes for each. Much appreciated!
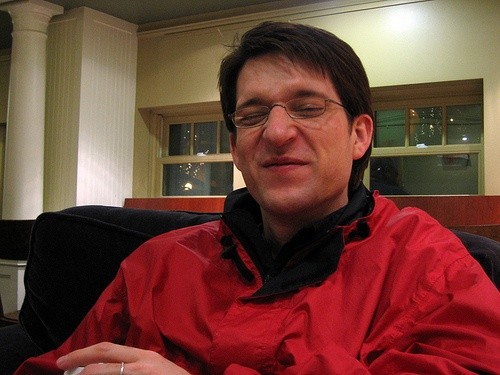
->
[0,205,500,375]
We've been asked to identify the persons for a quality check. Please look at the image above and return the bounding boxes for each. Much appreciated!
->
[14,21,500,375]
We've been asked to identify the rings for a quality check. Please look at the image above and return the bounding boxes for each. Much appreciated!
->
[120,361,125,374]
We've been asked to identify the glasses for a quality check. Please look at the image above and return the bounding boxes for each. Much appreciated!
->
[226,95,346,129]
